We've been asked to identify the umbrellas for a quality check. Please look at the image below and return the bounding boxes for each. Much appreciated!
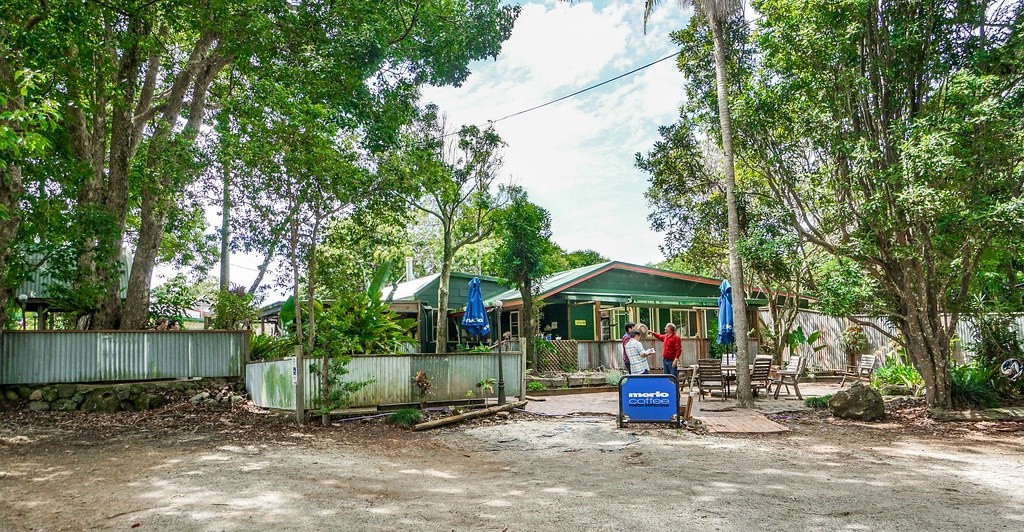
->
[461,277,490,350]
[717,279,734,396]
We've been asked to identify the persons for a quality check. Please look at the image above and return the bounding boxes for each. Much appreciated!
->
[149,317,180,330]
[543,332,551,340]
[622,323,656,375]
[648,323,681,379]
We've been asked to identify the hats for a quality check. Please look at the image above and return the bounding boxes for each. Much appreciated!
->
[544,325,553,331]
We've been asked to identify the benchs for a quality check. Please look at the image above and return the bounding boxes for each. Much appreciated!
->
[842,353,876,387]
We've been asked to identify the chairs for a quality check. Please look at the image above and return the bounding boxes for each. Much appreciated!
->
[675,351,806,400]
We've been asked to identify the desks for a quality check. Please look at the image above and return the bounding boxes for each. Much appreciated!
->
[689,365,756,394]
